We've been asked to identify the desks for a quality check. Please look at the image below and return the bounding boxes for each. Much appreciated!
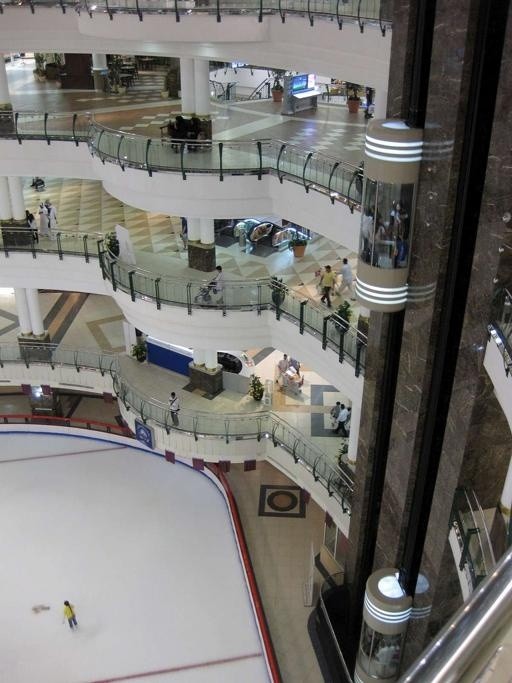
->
[118,58,158,89]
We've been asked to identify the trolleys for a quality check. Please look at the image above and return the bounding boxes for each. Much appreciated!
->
[318,273,336,296]
[286,377,301,395]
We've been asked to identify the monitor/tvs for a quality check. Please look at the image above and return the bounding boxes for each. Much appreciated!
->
[292,75,308,92]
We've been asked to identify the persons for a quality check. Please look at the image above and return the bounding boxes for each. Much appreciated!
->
[212,265,225,304]
[360,198,409,269]
[274,353,290,383]
[334,257,355,301]
[289,357,302,378]
[63,600,77,630]
[179,216,187,249]
[330,400,352,437]
[319,265,338,307]
[26,197,58,237]
[168,392,180,426]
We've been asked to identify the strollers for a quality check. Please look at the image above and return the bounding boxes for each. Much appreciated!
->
[195,276,214,304]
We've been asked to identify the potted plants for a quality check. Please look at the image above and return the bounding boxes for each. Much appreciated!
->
[330,299,353,335]
[269,276,287,305]
[132,341,147,363]
[288,238,308,257]
[270,68,286,102]
[345,82,364,113]
[105,231,120,259]
[337,444,348,470]
[249,374,264,401]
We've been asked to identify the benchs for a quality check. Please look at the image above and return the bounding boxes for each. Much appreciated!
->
[159,121,211,149]
